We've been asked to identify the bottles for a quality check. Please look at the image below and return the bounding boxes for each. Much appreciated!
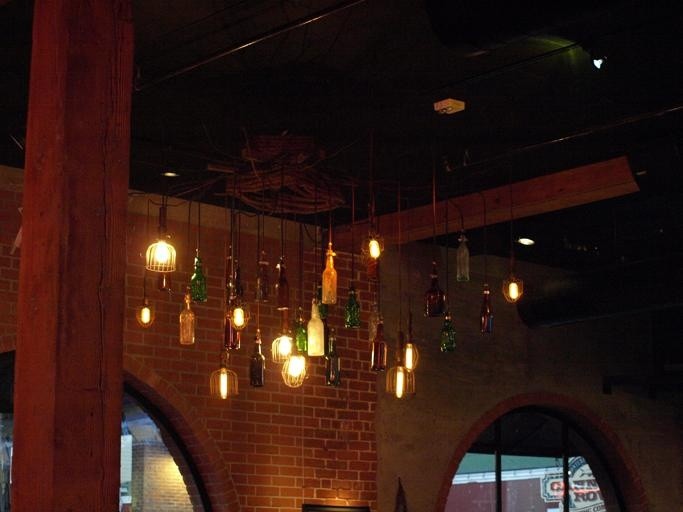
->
[158,258,208,346]
[424,235,493,354]
[221,241,389,387]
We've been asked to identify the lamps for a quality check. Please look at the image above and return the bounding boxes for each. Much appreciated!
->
[226,173,250,331]
[271,177,297,364]
[401,184,420,374]
[280,213,310,389]
[386,192,418,398]
[502,154,522,305]
[136,229,155,328]
[208,255,239,401]
[367,188,382,261]
[144,156,177,274]
